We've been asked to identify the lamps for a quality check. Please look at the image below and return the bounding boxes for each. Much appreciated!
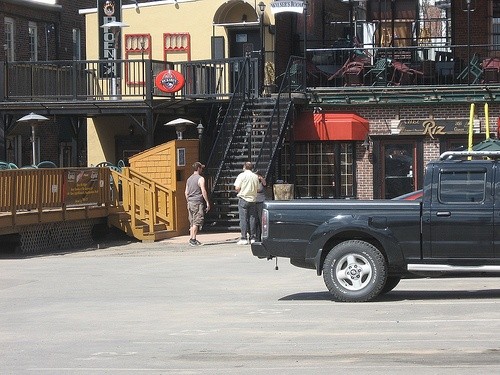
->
[129,124,137,136]
[258,2,266,11]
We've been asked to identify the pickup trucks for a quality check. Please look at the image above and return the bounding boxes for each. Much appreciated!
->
[249,151,500,303]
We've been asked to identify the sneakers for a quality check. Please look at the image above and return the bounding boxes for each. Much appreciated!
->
[237,240,248,245]
[249,239,255,244]
[189,239,204,246]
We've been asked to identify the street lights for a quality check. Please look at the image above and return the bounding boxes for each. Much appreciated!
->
[16,112,49,169]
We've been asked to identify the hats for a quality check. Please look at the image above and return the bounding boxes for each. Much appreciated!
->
[192,162,205,169]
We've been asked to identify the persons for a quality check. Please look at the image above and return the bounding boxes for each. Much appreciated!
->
[185,162,210,246]
[255,176,266,223]
[234,161,259,245]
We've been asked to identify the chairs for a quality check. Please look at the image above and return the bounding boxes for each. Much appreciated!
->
[276,37,500,92]
[0,159,124,203]
